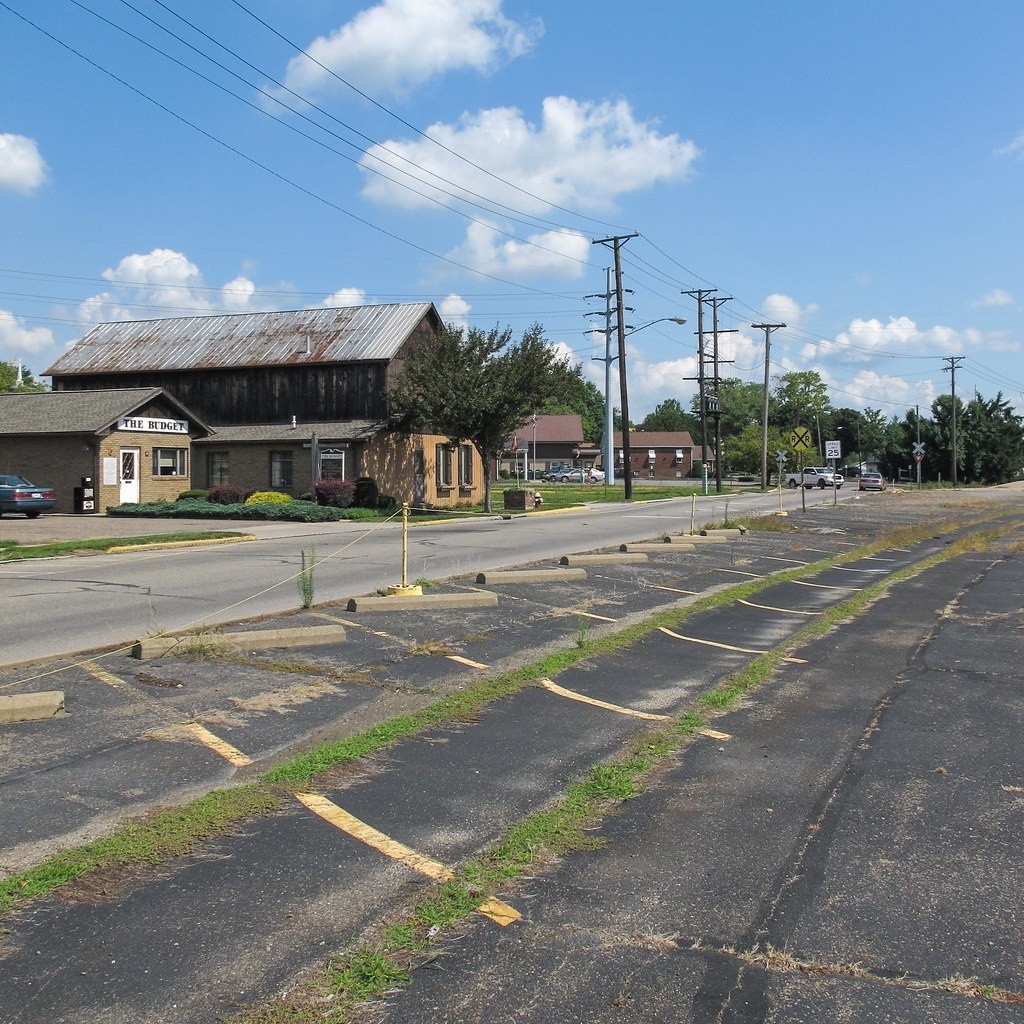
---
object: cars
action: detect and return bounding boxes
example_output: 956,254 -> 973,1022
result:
859,473 -> 887,491
0,475 -> 57,520
542,467 -> 606,484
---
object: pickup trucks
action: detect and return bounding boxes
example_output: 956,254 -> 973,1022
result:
784,467 -> 845,489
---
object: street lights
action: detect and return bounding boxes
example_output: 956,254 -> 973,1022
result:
617,316 -> 688,499
